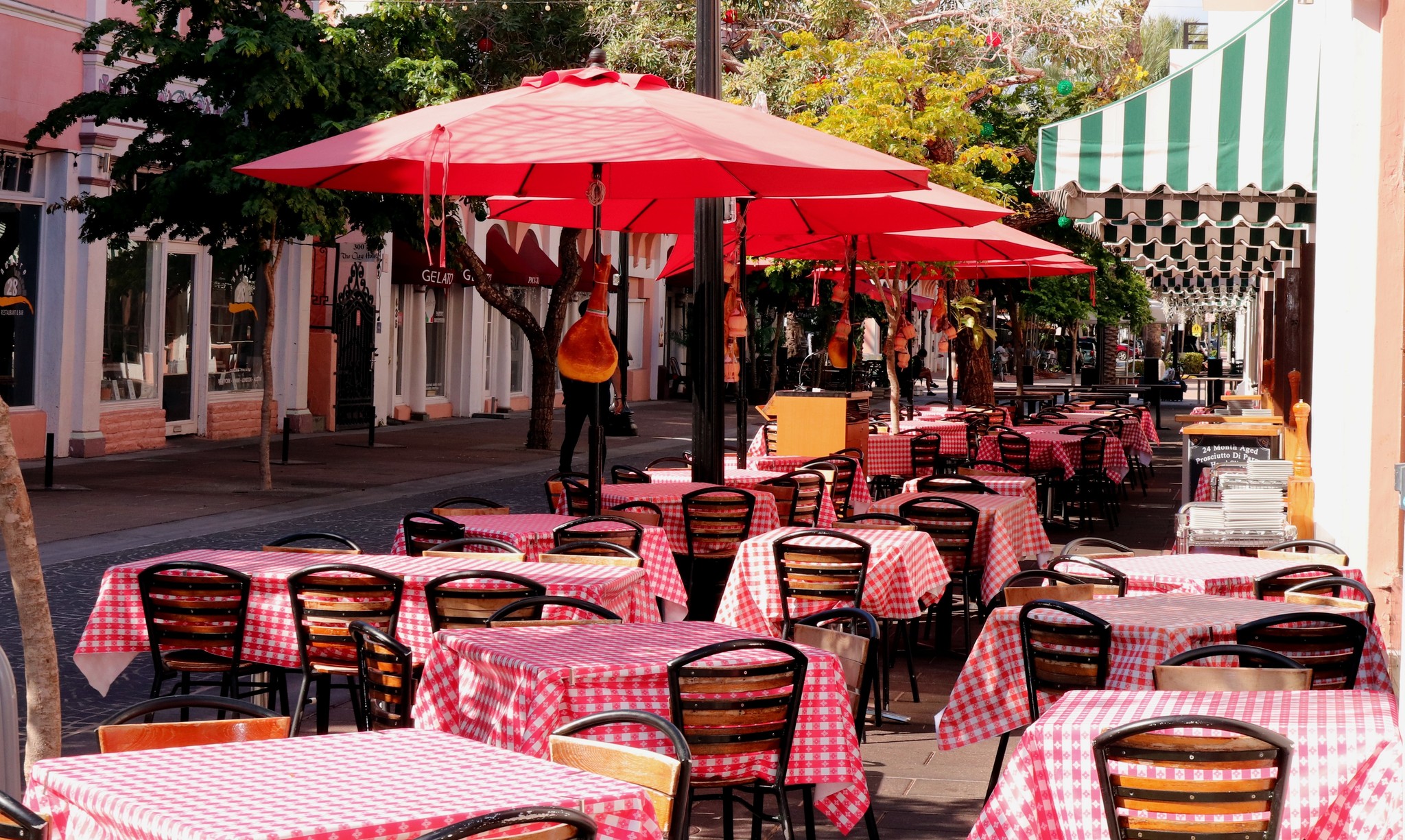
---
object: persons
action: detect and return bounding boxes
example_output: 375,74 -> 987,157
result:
1213,340 -> 1218,348
995,341 -> 1058,375
910,348 -> 938,396
558,298 -> 623,477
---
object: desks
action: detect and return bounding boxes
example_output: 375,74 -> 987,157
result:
763,384 -> 1181,534
554,483 -> 780,554
390,515 -> 688,622
73,549 -> 662,696
714,526 -> 952,710
1190,373 -> 1244,408
1042,553 -> 1389,669
862,492 -> 1053,606
627,469 -> 837,527
966,689 -> 1405,840
934,592 -> 1394,751
24,729 -> 665,840
411,621 -> 871,840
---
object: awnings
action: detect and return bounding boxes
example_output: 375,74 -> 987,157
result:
558,244 -> 595,291
1031,0 -> 1320,307
486,226 -> 540,287
442,227 -> 493,285
666,245 -> 694,288
518,233 -> 562,285
392,230 -> 454,286
585,245 -> 629,294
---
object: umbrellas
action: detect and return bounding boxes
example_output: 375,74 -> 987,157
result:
742,251 -> 1082,413
484,175 -> 1015,473
233,46 -> 934,513
685,222 -> 1071,391
806,263 -> 1098,411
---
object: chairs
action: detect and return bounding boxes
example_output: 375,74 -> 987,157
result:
670,357 -> 689,397
984,538 -> 1376,840
99,464 -> 986,840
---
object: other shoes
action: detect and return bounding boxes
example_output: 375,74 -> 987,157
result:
930,383 -> 939,388
927,391 -> 936,396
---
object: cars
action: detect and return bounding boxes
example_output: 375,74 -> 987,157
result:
1116,339 -> 1144,361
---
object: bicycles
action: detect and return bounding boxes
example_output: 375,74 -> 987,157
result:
990,351 -> 1005,382
1037,353 -> 1060,373
1164,349 -> 1191,379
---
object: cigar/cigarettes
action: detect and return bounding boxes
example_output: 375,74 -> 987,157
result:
617,413 -> 621,414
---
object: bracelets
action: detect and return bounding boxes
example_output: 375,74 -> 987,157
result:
615,398 -> 621,400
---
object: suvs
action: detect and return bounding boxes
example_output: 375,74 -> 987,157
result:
1055,336 -> 1097,374
1199,341 -> 1208,362
